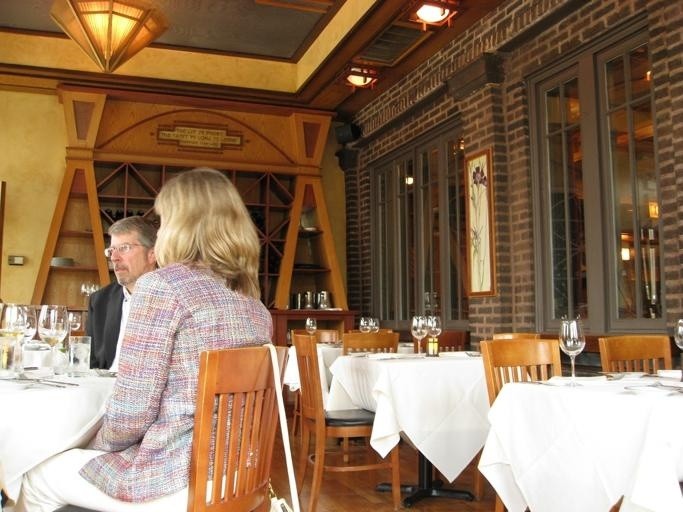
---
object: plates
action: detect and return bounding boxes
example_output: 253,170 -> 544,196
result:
465,351 -> 481,358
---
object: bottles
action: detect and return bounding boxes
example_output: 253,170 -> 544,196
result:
290,291 -> 331,309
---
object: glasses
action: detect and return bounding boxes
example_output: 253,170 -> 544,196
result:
104,243 -> 143,257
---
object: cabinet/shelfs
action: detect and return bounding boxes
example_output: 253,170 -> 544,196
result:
28,158 -> 349,311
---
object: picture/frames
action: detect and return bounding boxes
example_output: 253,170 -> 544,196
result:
461,148 -> 497,299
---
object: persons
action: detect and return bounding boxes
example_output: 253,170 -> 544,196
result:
2,168 -> 274,512
69,216 -> 160,370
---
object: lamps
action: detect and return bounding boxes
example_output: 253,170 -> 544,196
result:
342,65 -> 379,94
392,0 -> 467,33
48,1 -> 171,74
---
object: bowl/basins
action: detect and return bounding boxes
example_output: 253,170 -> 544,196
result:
49,256 -> 73,267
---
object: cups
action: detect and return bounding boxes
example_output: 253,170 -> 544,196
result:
359,316 -> 379,333
304,317 -> 317,335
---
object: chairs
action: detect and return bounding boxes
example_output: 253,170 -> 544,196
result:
0,315 -> 682,512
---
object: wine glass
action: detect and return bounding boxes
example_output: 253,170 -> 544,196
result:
672,318 -> 683,382
411,316 -> 428,360
559,320 -> 586,387
0,302 -> 91,387
427,315 -> 441,357
